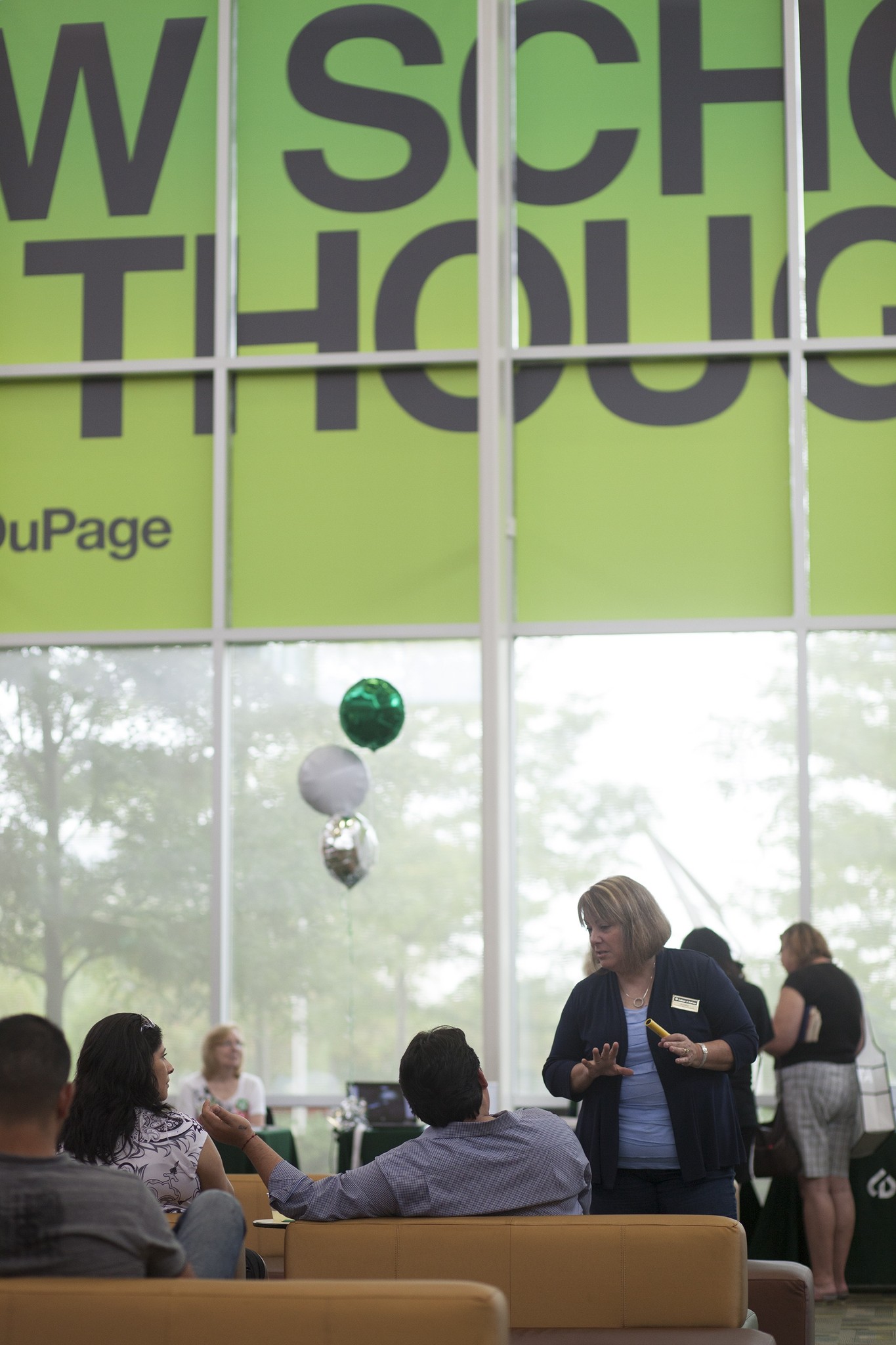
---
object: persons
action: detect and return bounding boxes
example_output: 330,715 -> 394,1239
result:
159,1023 -> 268,1133
540,875 -> 761,1220
761,921 -> 867,1302
680,927 -> 775,1258
200,1023 -> 594,1224
1,1012 -> 247,1280
57,1010 -> 269,1282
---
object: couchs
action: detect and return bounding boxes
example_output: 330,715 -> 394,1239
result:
0,1173 -> 815,1345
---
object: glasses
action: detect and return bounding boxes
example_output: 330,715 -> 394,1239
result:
140,1013 -> 155,1032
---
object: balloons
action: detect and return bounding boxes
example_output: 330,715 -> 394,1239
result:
321,809 -> 378,891
335,676 -> 403,751
295,742 -> 368,816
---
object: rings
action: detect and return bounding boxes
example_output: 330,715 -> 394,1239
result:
681,1048 -> 689,1056
594,1058 -> 602,1064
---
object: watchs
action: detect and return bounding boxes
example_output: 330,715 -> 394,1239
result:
694,1042 -> 708,1068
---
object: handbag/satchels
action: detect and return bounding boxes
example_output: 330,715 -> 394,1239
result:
744,1102 -> 802,1180
799,1004 -> 822,1044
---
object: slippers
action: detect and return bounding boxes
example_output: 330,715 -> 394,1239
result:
835,1289 -> 850,1301
814,1283 -> 838,1302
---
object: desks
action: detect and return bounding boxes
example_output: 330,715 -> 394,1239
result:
749,1129 -> 896,1295
210,1125 -> 301,1175
333,1125 -> 425,1175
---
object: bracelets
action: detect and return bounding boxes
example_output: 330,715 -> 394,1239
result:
241,1133 -> 259,1151
